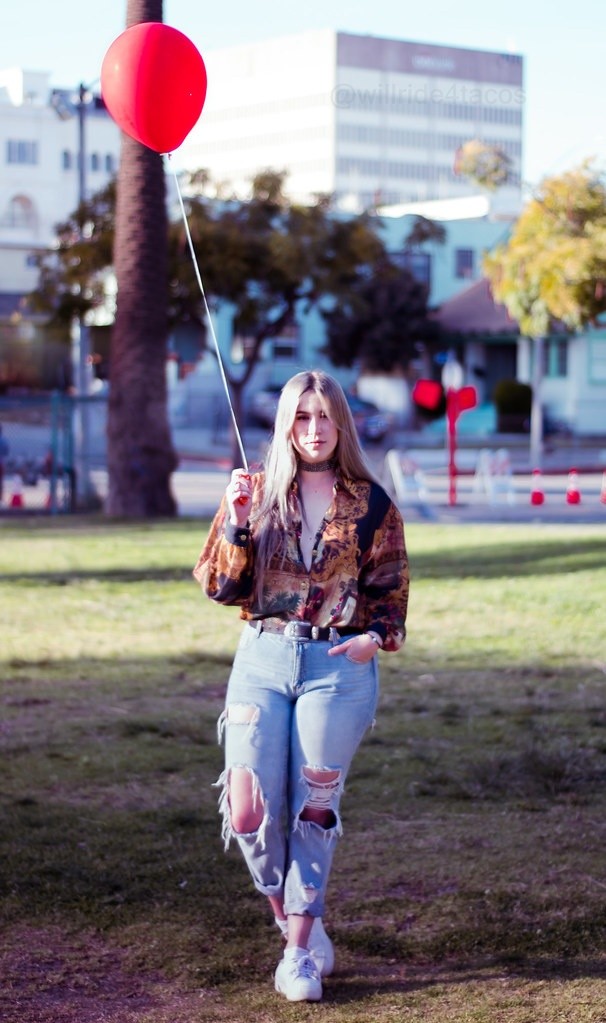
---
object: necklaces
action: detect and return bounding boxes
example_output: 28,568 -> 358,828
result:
296,456 -> 337,472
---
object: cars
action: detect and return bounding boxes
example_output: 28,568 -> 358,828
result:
254,380 -> 392,442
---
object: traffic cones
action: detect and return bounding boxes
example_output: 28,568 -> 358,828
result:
566,468 -> 579,505
530,468 -> 545,506
601,471 -> 606,504
12,473 -> 25,508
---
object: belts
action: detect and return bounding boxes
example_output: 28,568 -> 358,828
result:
248,620 -> 365,642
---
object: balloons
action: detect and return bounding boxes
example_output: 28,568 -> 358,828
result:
100,21 -> 208,152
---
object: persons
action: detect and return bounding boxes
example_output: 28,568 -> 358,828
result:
192,368 -> 410,1003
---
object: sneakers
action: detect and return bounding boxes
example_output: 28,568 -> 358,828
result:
274,911 -> 335,1001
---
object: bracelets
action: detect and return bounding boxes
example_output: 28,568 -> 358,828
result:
365,631 -> 381,647
